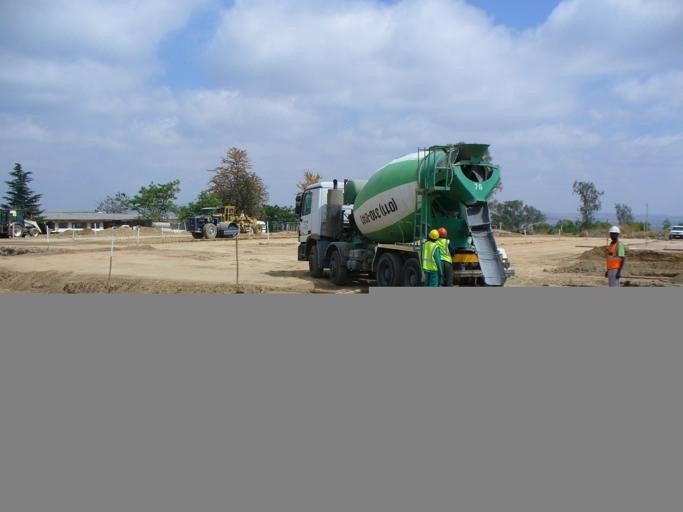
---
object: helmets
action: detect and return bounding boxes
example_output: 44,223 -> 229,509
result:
427,229 -> 439,241
607,225 -> 620,234
436,227 -> 448,238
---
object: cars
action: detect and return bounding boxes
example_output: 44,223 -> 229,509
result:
669,226 -> 683,240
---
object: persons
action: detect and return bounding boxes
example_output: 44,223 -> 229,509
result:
421,228 -> 444,287
603,225 -> 625,288
436,227 -> 455,286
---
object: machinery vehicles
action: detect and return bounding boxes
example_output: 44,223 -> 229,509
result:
296,143 -> 515,287
1,208 -> 42,237
187,206 -> 262,239
